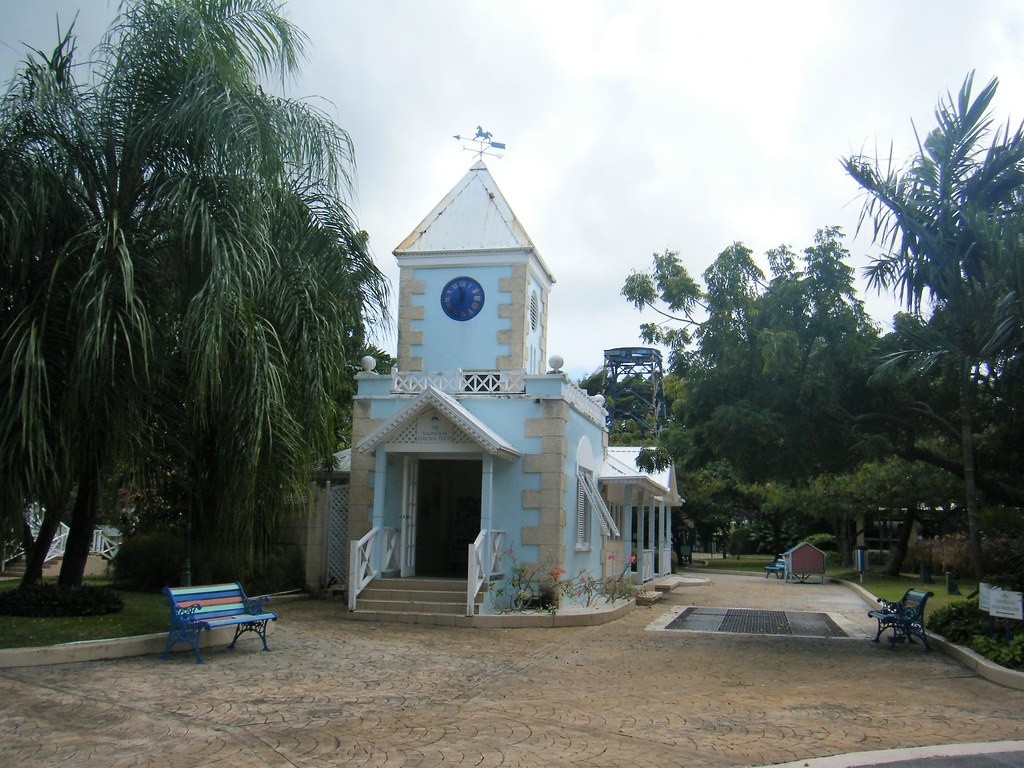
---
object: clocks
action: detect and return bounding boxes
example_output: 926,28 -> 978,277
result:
441,276 -> 485,321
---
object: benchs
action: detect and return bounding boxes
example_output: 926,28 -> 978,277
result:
867,588 -> 934,651
764,558 -> 785,579
160,582 -> 278,665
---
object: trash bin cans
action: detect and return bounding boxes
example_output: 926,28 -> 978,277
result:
853,546 -> 869,572
